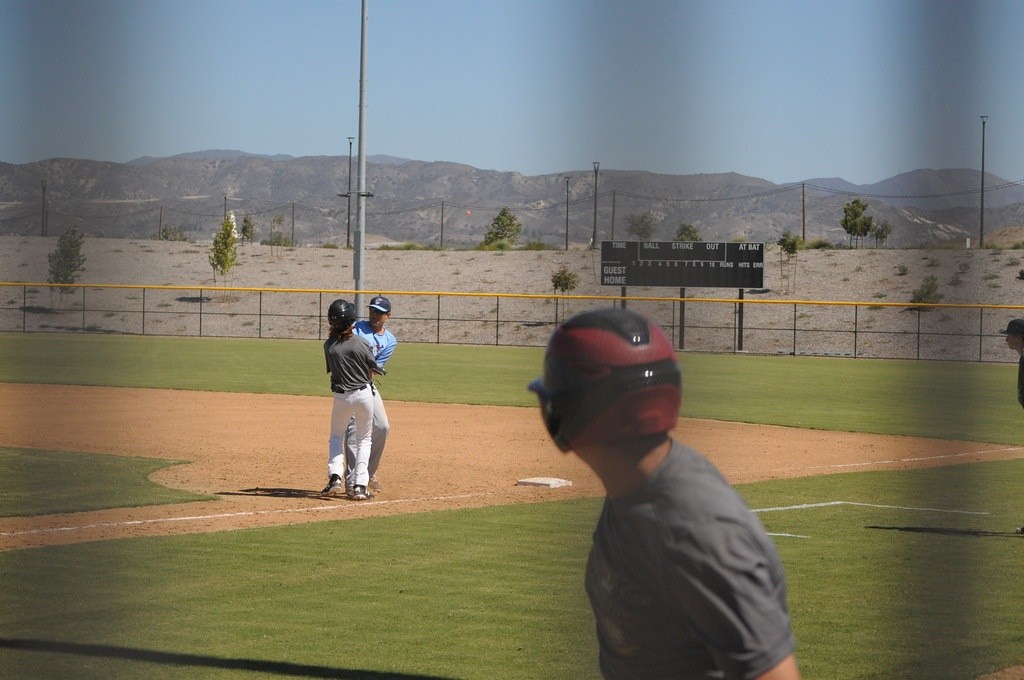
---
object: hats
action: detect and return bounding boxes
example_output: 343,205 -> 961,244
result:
366,297 -> 391,312
1000,319 -> 1024,335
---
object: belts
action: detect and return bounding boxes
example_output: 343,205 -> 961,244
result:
338,385 -> 367,393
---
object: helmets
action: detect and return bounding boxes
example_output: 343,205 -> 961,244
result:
528,307 -> 682,452
328,299 -> 356,329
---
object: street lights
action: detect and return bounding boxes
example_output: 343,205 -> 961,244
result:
593,160 -> 601,250
346,136 -> 356,249
564,176 -> 572,250
979,115 -> 989,248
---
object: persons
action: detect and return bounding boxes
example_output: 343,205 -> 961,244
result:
998,319 -> 1024,536
526,307 -> 803,680
322,296 -> 397,501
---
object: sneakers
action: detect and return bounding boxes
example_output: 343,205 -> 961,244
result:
369,475 -> 380,488
346,486 -> 355,497
320,474 -> 342,496
352,484 -> 373,500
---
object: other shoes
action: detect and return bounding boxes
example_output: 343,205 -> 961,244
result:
1016,528 -> 1024,534
